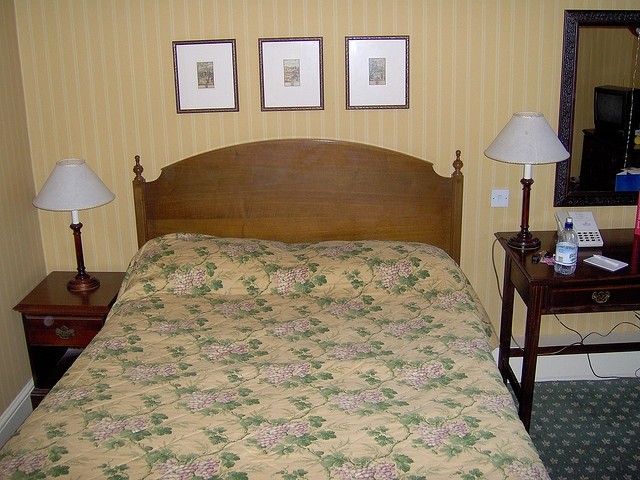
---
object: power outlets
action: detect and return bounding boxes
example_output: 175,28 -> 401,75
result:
490,189 -> 510,208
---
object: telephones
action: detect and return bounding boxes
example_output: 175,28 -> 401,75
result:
554,211 -> 604,248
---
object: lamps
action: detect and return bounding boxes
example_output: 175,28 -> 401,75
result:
483,110 -> 571,254
32,157 -> 116,295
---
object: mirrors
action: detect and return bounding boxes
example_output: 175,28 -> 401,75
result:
553,9 -> 640,206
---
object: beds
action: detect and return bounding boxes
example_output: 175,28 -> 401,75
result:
0,137 -> 551,479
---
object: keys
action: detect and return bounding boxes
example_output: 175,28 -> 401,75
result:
531,250 -> 545,264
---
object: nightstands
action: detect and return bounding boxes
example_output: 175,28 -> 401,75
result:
12,270 -> 126,411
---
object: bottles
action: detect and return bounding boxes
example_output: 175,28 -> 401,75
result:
554,217 -> 579,276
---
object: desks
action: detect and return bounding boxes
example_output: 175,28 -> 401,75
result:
494,228 -> 640,435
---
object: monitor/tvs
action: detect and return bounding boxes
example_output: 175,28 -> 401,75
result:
593,84 -> 640,131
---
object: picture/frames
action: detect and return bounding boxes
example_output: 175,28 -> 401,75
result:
344,34 -> 410,109
171,39 -> 240,114
258,36 -> 326,112
